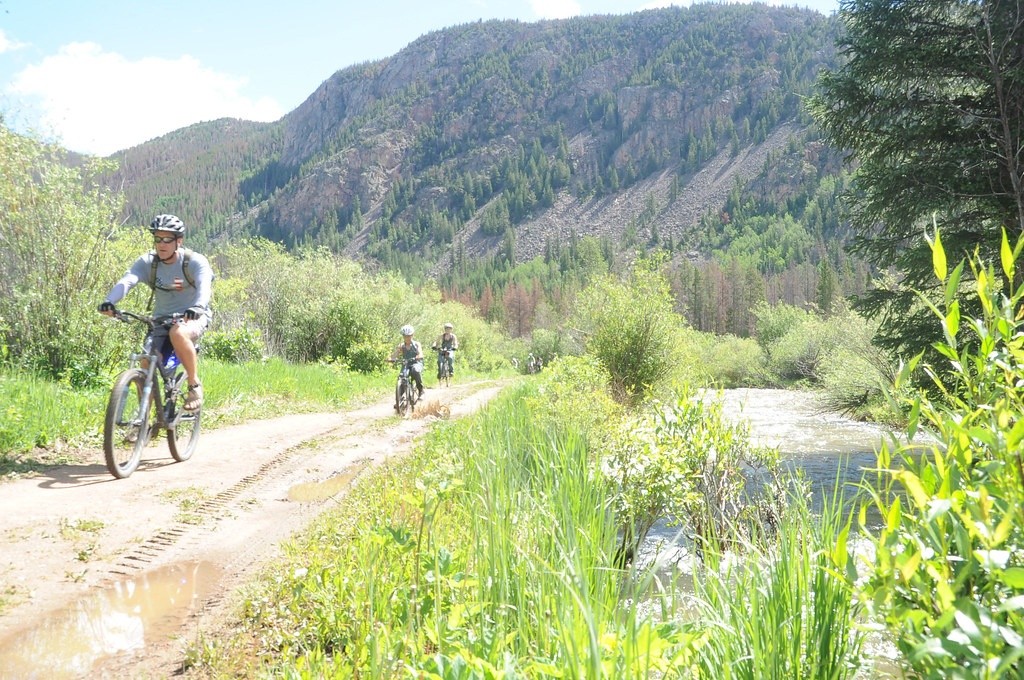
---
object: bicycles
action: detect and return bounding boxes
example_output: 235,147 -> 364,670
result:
434,346 -> 456,389
104,309 -> 202,480
388,357 -> 426,418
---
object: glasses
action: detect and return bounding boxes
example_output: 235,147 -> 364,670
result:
154,236 -> 178,244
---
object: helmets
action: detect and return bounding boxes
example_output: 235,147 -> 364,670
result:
444,323 -> 453,329
147,213 -> 186,238
399,325 -> 415,336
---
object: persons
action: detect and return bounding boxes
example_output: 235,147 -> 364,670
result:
386,325 -> 424,408
433,323 -> 457,379
511,353 -> 543,374
98,214 -> 213,443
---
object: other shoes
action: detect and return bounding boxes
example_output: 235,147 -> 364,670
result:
419,388 -> 424,396
393,404 -> 397,409
437,373 -> 442,379
449,371 -> 454,377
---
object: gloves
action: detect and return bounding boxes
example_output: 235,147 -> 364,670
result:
451,348 -> 456,351
431,347 -> 437,351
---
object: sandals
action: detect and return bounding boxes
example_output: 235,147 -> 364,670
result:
183,382 -> 203,412
128,427 -> 140,443
